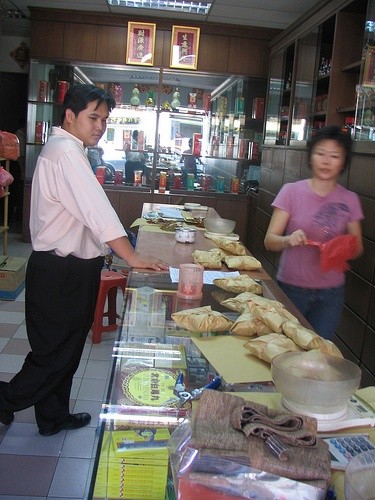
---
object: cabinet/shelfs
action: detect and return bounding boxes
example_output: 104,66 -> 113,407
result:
0,155 -> 10,256
247,0 -> 375,390
21,55 -> 267,245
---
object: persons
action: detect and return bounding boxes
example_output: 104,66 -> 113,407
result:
263,125 -> 364,340
0,83 -> 170,436
180,138 -> 203,183
125,130 -> 148,181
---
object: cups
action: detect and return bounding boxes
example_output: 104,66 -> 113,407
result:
177,264 -> 205,300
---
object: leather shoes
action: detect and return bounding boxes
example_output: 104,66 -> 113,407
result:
39,412 -> 91,436
0,380 -> 15,426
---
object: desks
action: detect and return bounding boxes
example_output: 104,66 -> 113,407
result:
145,162 -> 202,182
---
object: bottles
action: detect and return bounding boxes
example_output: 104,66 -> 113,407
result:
170,88 -> 180,109
130,84 -> 140,106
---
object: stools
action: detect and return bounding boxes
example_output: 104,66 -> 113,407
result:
90,270 -> 127,344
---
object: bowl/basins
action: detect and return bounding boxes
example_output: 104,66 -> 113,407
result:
344,449 -> 375,500
175,226 -> 197,244
269,350 -> 361,416
203,217 -> 236,234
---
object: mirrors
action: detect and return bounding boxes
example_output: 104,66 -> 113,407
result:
25,59 -> 245,191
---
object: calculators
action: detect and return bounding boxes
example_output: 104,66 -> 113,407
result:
324,435 -> 375,470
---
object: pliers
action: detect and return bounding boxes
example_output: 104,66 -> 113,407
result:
164,373 -> 220,409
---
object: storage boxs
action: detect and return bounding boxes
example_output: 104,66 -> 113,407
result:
37,79 -> 123,105
35,121 -> 46,143
107,128 -> 244,160
0,254 -> 29,300
186,91 -> 245,120
251,98 -> 265,120
97,165 -> 240,194
248,142 -> 259,160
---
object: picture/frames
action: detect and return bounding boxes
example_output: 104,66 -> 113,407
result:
125,22 -> 156,66
169,25 -> 201,71
